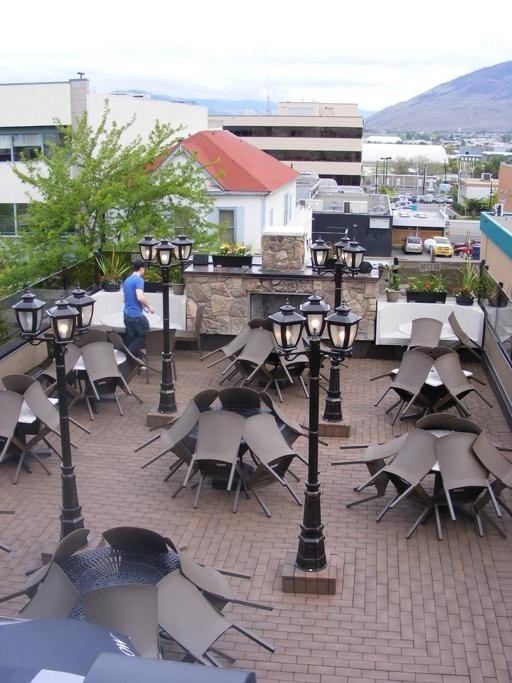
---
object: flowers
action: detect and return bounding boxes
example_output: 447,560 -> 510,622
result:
406,274 -> 446,293
212,243 -> 250,257
143,272 -> 162,283
453,287 -> 476,299
93,249 -> 131,281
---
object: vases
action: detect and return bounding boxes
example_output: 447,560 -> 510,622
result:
144,282 -> 160,293
172,268 -> 185,295
456,296 -> 474,306
102,279 -> 121,292
406,289 -> 447,304
211,255 -> 253,269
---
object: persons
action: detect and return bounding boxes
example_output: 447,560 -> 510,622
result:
124,259 -> 155,356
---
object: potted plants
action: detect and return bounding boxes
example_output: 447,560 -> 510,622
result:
381,264 -> 401,302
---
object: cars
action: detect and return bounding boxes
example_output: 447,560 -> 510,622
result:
390,190 -> 455,209
431,235 -> 452,257
454,241 -> 482,258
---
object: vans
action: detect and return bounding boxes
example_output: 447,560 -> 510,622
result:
401,235 -> 424,253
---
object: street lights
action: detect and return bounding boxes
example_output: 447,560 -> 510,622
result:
137,232 -> 193,412
271,291 -> 363,573
380,155 -> 393,187
311,231 -> 366,422
12,286 -> 97,550
